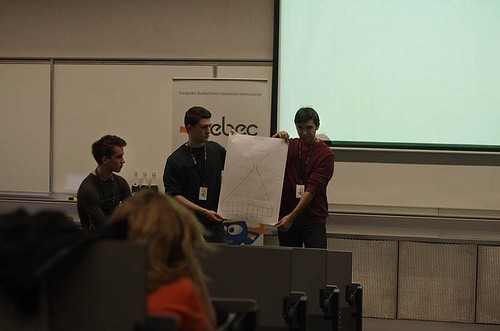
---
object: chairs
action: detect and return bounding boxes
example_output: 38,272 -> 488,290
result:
0,242 -> 363,331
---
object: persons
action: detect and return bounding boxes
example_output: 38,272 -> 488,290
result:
162,106 -> 239,244
0,188 -> 218,331
77,135 -> 133,240
271,107 -> 334,249
